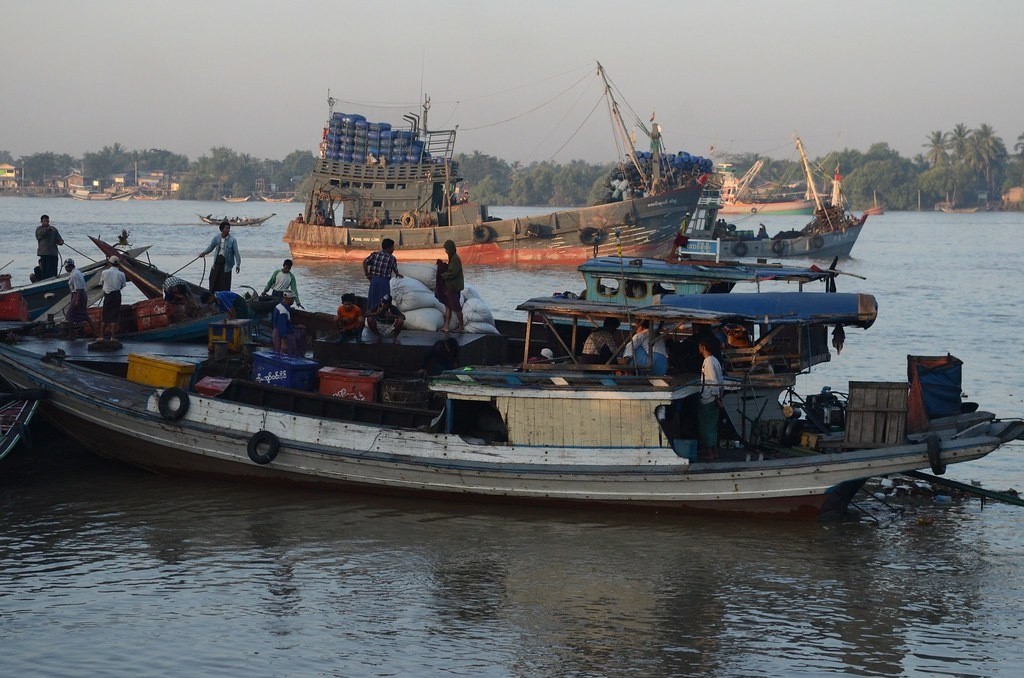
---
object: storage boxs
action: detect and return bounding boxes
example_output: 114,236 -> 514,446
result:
208,319 -> 252,354
0,292 -> 28,321
251,349 -> 320,393
840,381 -> 910,448
126,352 -> 196,391
316,366 -> 384,403
132,297 -> 169,332
84,304 -> 134,337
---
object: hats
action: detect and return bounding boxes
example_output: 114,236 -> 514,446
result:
381,294 -> 392,304
109,256 -> 119,264
61,258 -> 74,266
541,348 -> 555,364
283,290 -> 296,299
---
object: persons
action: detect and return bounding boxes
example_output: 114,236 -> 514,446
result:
199,222 -> 241,291
758,223 -> 765,237
435,240 -> 464,332
207,214 -> 212,218
273,291 -> 295,352
363,238 -> 404,309
61,258 -> 99,340
295,213 -> 303,223
200,291 -> 249,319
581,318 -> 624,375
313,206 -> 325,225
224,216 -> 227,221
682,323 -> 722,373
96,255 -> 126,341
696,337 -> 724,461
336,294 -> 365,344
438,185 -> 444,210
364,294 -> 405,344
30,214 -> 64,283
460,192 -> 468,202
717,219 -> 727,230
623,319 -> 668,376
262,259 -> 301,307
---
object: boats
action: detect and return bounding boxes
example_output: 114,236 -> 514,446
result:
222,194 -> 251,203
677,128 -> 870,262
1,242 -> 156,324
67,184 -> 135,201
133,192 -> 164,200
1,235 -> 1024,519
260,195 -> 296,203
942,186 -> 979,212
292,66 -> 713,267
194,211 -> 277,227
864,189 -> 885,215
719,160 -> 848,215
0,387 -> 52,464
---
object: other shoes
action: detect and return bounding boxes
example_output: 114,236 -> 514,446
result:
95,337 -> 104,341
392,338 -> 401,344
111,337 -> 119,341
356,338 -> 365,344
336,336 -> 344,344
372,336 -> 382,343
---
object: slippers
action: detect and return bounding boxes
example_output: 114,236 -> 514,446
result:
452,327 -> 464,333
437,328 -> 449,332
